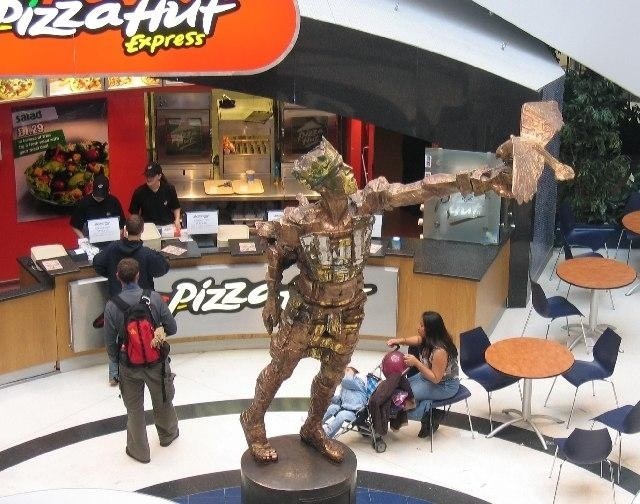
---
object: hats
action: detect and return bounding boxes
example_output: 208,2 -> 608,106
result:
142,161 -> 162,178
91,175 -> 109,198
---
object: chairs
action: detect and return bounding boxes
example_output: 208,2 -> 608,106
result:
549,428 -> 616,504
587,400 -> 640,484
459,326 -> 524,432
549,226 -> 615,281
406,344 -> 475,454
613,192 -> 640,264
544,326 -> 622,430
556,235 -> 616,310
521,268 -> 589,356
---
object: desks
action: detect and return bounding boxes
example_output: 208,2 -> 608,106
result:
555,257 -> 637,352
622,211 -> 640,295
484,336 -> 576,451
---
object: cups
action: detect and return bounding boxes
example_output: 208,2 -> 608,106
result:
240,170 -> 254,186
390,237 -> 402,252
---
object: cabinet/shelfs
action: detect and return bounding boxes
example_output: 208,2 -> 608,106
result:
210,88 -> 278,185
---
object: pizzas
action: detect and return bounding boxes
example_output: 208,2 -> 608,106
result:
0,79 -> 36,99
70,77 -> 102,92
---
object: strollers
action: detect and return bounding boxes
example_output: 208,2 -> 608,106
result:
333,339 -> 414,455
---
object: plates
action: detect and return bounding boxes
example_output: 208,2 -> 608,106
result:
25,141 -> 109,206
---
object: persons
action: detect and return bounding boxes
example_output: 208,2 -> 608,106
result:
129,162 -> 181,230
239,135 -> 515,463
387,312 -> 460,437
94,214 -> 170,385
321,366 -> 368,437
104,257 -> 179,462
69,174 -> 127,247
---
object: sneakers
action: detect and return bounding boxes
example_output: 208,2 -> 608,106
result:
416,409 -> 444,439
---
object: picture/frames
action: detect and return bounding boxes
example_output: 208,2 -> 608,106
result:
156,108 -> 211,166
0,78 -> 196,104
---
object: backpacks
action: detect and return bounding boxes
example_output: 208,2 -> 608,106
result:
108,287 -> 172,368
